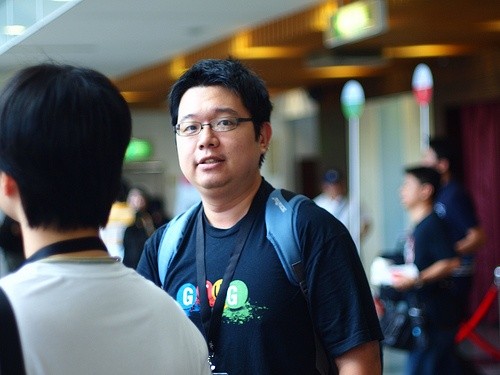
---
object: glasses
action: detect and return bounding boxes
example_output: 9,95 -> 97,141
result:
173,118 -> 253,136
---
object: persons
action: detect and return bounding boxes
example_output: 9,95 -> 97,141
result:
0,63 -> 213,375
310,168 -> 371,243
136,59 -> 385,375
392,165 -> 464,375
421,135 -> 486,375
99,179 -> 172,269
